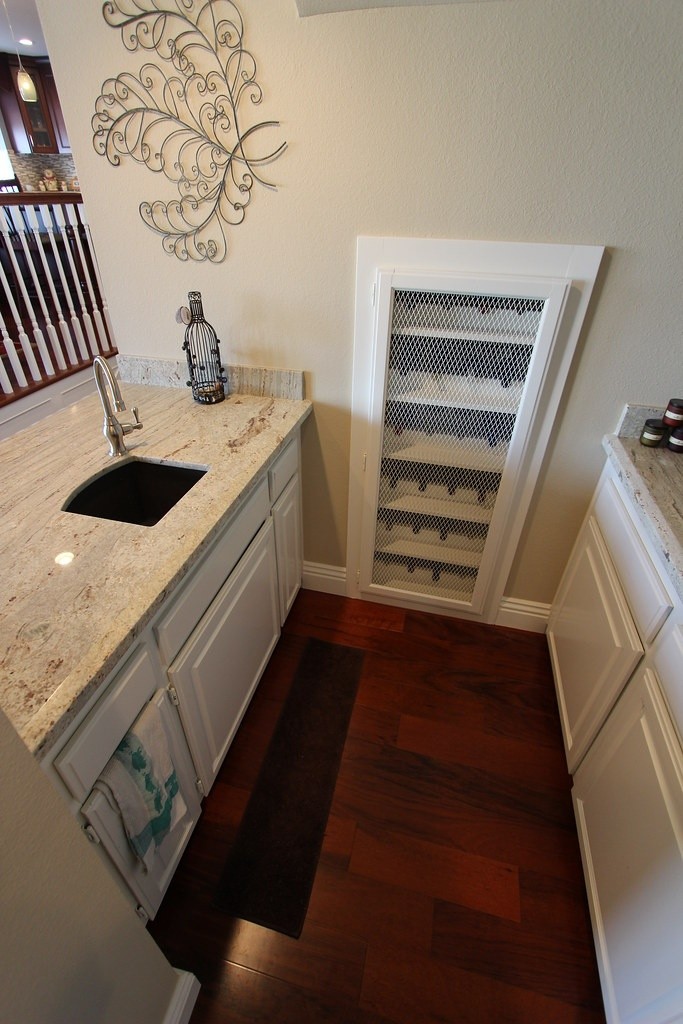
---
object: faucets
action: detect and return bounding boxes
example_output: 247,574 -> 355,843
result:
93,355 -> 144,458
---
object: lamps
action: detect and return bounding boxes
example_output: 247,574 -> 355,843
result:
2,0 -> 38,101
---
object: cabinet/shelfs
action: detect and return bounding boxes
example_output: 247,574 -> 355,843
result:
545,458 -> 683,1024
40,435 -> 307,925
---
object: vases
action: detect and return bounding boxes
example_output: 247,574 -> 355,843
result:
182,290 -> 225,405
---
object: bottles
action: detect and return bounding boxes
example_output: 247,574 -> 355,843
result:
640,419 -> 665,447
661,399 -> 683,427
666,427 -> 683,454
